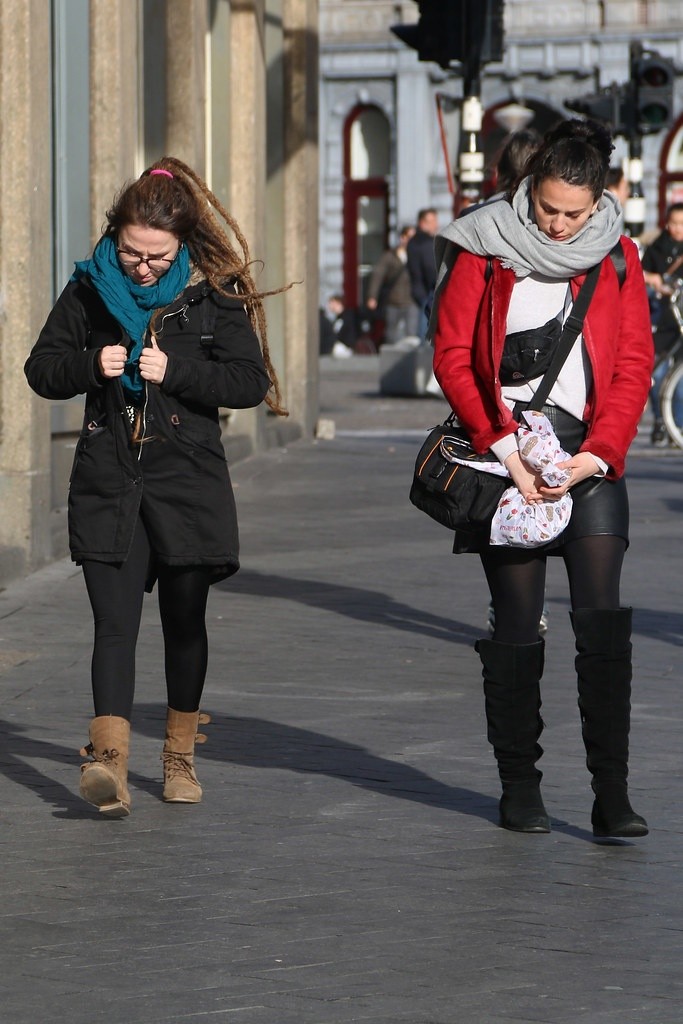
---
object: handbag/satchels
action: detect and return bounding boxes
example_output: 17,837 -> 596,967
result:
408,425 -> 515,533
499,282 -> 572,385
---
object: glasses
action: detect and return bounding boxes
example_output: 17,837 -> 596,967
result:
115,234 -> 182,273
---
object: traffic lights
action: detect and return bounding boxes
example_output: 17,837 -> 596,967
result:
634,59 -> 674,136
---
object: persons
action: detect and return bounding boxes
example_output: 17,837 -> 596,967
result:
604,168 -> 630,206
640,202 -> 683,447
23,157 -> 290,818
327,296 -> 357,358
457,130 -> 549,634
366,225 -> 418,342
407,208 -> 439,339
423,119 -> 655,838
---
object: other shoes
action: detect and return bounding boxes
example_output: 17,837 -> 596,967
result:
650,421 -> 669,447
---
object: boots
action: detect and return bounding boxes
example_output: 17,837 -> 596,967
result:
160,705 -> 210,803
569,605 -> 649,836
80,715 -> 132,819
475,633 -> 550,833
